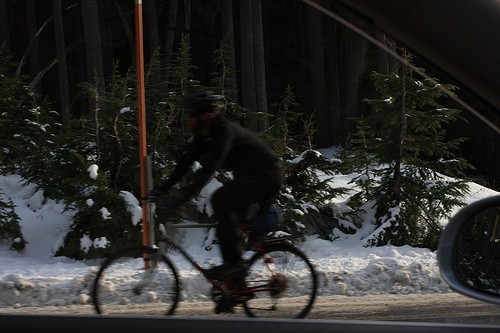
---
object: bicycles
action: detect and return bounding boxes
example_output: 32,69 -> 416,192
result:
89,195 -> 320,320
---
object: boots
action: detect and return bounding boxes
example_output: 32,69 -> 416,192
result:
214,269 -> 255,315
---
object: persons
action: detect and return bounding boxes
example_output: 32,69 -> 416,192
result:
148,86 -> 284,309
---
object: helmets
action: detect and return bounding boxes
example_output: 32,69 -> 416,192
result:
183,88 -> 227,120
